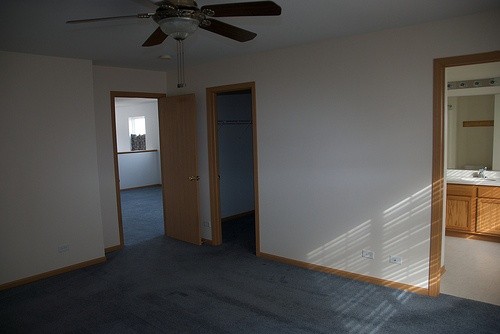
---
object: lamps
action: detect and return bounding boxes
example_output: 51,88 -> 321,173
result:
157,0 -> 202,89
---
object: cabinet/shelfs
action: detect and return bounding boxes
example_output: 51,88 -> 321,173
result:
445,183 -> 500,243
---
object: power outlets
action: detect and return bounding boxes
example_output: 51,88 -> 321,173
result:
362,249 -> 374,258
389,256 -> 402,264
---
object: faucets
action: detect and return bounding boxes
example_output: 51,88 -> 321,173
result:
478,167 -> 487,178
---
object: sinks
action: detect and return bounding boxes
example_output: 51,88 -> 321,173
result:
461,178 -> 497,182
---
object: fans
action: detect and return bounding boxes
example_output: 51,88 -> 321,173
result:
65,0 -> 282,47
